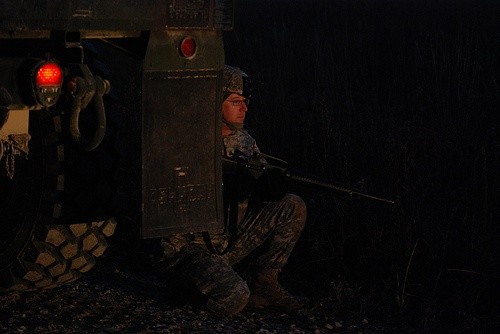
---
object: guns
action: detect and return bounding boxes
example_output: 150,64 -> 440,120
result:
220,146 -> 394,204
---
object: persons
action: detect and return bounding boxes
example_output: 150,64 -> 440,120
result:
159,65 -> 307,317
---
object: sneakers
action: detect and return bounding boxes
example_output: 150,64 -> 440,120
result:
249,278 -> 308,310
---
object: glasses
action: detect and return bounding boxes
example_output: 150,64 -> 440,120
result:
223,98 -> 250,106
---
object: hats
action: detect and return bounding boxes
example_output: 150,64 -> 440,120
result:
221,65 -> 251,95
